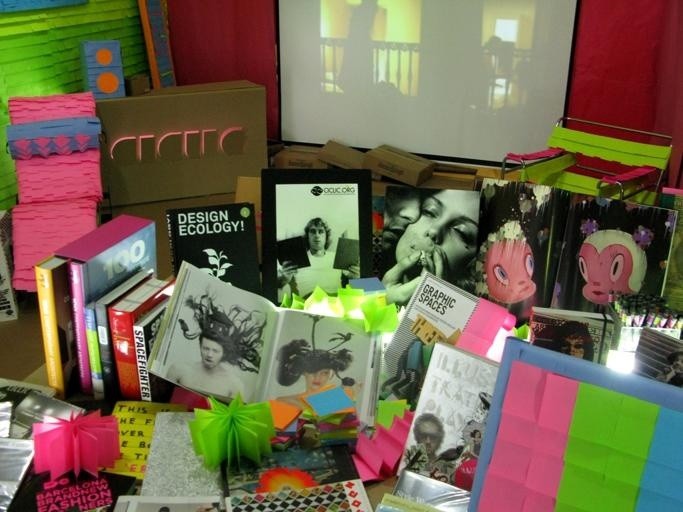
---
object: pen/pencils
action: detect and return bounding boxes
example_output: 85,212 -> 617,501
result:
608,289 -> 683,329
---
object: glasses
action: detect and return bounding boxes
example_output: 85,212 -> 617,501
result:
416,431 -> 439,443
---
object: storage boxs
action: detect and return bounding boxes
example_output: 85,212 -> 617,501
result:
96,80 -> 268,207
111,194 -> 261,278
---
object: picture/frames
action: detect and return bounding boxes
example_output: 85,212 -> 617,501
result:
261,168 -> 373,302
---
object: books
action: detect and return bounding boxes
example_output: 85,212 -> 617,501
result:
0,170 -> 682,512
